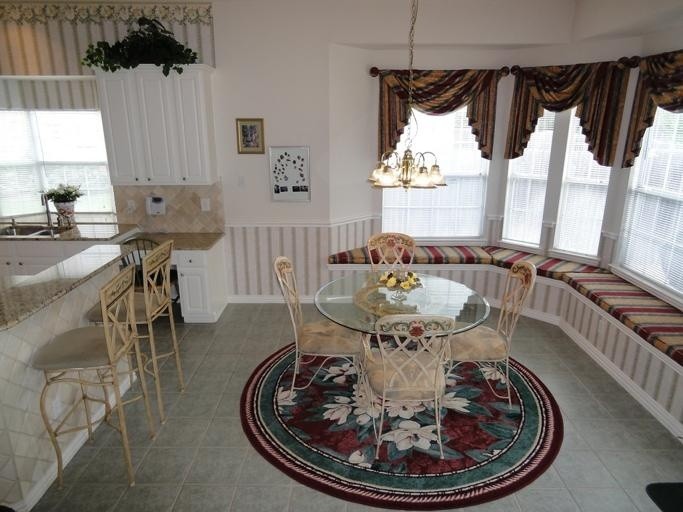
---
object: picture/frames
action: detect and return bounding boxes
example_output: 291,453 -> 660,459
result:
236,119 -> 265,154
269,145 -> 312,203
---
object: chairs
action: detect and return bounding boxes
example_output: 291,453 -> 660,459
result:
31,265 -> 154,489
273,256 -> 363,403
362,314 -> 454,461
86,239 -> 185,424
445,261 -> 536,410
367,232 -> 414,271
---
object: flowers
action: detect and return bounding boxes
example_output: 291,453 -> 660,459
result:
44,184 -> 83,203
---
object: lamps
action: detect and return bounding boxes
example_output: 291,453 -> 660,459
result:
368,0 -> 446,188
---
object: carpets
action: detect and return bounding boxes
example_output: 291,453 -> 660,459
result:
647,483 -> 683,512
240,341 -> 564,510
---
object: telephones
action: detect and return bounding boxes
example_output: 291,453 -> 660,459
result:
145,196 -> 165,215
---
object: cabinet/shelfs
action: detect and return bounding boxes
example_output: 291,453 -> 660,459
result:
95,64 -> 219,186
176,239 -> 227,324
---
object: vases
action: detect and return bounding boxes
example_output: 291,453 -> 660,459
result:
54,201 -> 74,218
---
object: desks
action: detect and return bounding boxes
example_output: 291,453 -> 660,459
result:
314,270 -> 490,350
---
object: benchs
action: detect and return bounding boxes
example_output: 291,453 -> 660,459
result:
328,246 -> 683,443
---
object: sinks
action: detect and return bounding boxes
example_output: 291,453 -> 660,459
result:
0,225 -> 44,241
31,226 -> 73,241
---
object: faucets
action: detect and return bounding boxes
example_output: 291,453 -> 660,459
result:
40,193 -> 53,226
